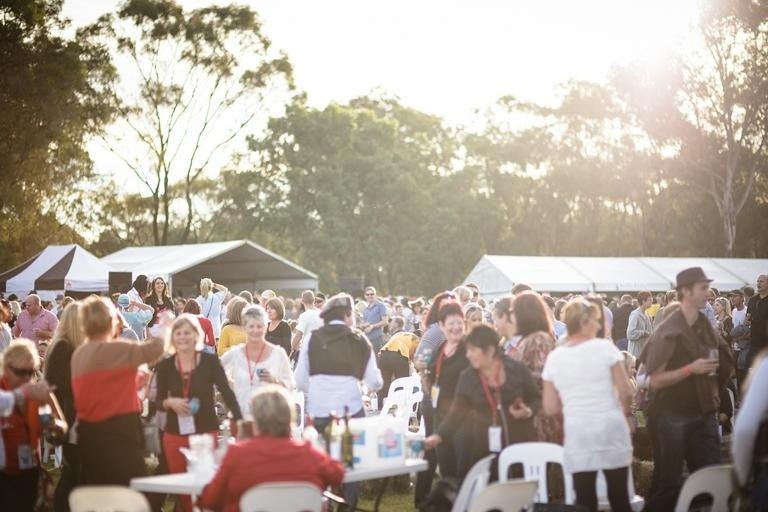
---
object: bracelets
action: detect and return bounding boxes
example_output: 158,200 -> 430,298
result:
625,411 -> 632,417
681,365 -> 691,377
14,389 -> 25,405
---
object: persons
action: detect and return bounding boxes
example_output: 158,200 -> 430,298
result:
1,267 -> 768,512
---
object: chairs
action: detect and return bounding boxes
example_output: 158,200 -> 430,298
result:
42,372 -> 741,512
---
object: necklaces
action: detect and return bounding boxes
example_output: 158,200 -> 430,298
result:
245,342 -> 267,386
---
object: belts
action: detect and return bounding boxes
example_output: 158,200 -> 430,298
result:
707,349 -> 720,377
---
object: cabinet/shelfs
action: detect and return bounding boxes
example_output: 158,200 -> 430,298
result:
370,389 -> 378,416
304,405 -> 354,465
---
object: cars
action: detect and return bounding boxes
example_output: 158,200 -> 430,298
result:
421,346 -> 432,367
256,368 -> 265,380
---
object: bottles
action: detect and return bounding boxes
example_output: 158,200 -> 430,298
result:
319,292 -> 354,317
728,289 -> 743,296
673,268 -> 713,288
55,295 -> 63,300
118,294 -> 130,308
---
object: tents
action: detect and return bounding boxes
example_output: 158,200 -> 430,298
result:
462,255 -> 768,307
99,239 -> 319,315
1,244 -> 133,316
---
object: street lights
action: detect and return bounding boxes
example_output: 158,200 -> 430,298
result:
135,414 -> 161,454
34,440 -> 65,512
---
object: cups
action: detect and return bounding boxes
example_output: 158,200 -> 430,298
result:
8,363 -> 36,377
